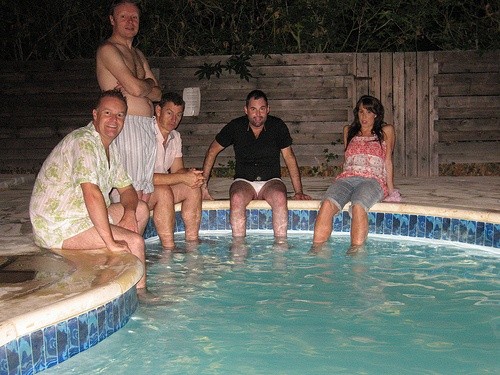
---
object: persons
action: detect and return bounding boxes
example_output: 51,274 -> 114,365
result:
304,97 -> 402,256
96,0 -> 163,199
200,90 -> 313,257
141,90 -> 207,253
29,90 -> 150,304
